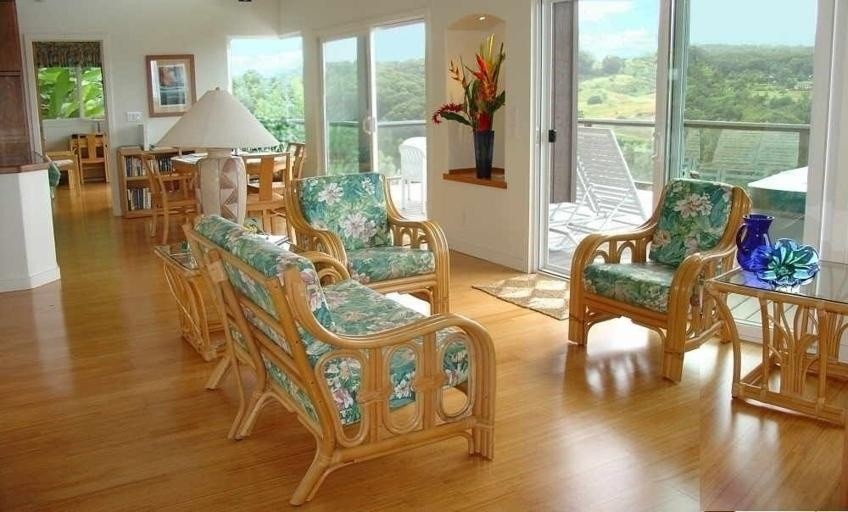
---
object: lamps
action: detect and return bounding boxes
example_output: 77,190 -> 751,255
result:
151,86 -> 279,228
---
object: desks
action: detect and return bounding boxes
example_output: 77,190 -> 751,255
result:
702,259 -> 848,425
68,134 -> 108,185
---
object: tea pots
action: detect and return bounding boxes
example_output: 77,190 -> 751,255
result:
735,213 -> 775,273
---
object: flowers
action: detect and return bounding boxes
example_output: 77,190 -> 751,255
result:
432,39 -> 507,131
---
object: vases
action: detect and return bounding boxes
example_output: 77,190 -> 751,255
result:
473,132 -> 495,181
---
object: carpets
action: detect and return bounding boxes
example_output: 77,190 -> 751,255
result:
470,270 -> 570,320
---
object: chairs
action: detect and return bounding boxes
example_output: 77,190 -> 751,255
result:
44,148 -> 84,200
182,171 -> 496,507
78,132 -> 110,184
136,141 -> 307,246
568,177 -> 752,382
547,127 -> 650,247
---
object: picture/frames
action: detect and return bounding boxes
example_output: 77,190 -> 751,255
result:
144,53 -> 197,119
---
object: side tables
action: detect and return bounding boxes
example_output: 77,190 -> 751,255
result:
152,240 -> 306,364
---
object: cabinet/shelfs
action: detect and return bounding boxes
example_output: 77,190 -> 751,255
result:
116,146 -> 199,220
